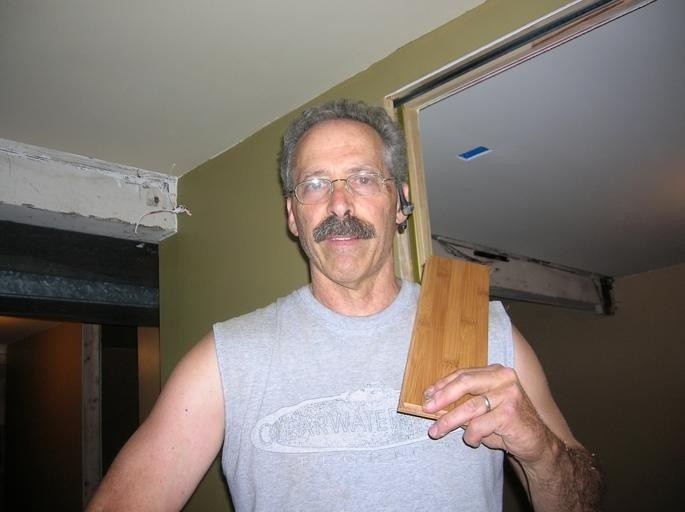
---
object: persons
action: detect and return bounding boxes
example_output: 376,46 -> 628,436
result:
88,101 -> 607,512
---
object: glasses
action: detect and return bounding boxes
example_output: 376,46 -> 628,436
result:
288,172 -> 397,205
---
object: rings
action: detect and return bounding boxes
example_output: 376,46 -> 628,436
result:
479,394 -> 490,411
